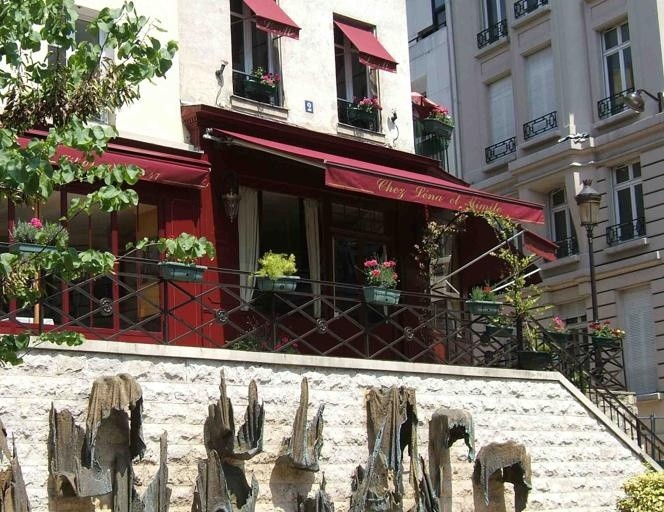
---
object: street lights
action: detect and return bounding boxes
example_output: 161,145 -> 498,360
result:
573,178 -> 605,387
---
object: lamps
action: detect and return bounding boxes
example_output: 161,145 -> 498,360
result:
622,89 -> 664,114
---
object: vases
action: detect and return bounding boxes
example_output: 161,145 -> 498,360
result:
363,286 -> 403,305
545,330 -> 571,344
465,300 -> 503,315
8,242 -> 61,260
592,337 -> 621,348
424,119 -> 455,139
348,109 -> 378,122
244,80 -> 276,98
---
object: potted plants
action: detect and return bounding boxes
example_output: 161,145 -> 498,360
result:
254,250 -> 301,293
518,322 -> 550,370
125,232 -> 216,283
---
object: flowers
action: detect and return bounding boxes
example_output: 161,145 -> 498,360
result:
362,253 -> 402,289
543,314 -> 571,332
252,66 -> 281,88
350,94 -> 382,114
589,319 -> 626,339
8,218 -> 70,246
427,104 -> 453,126
467,280 -> 499,301
